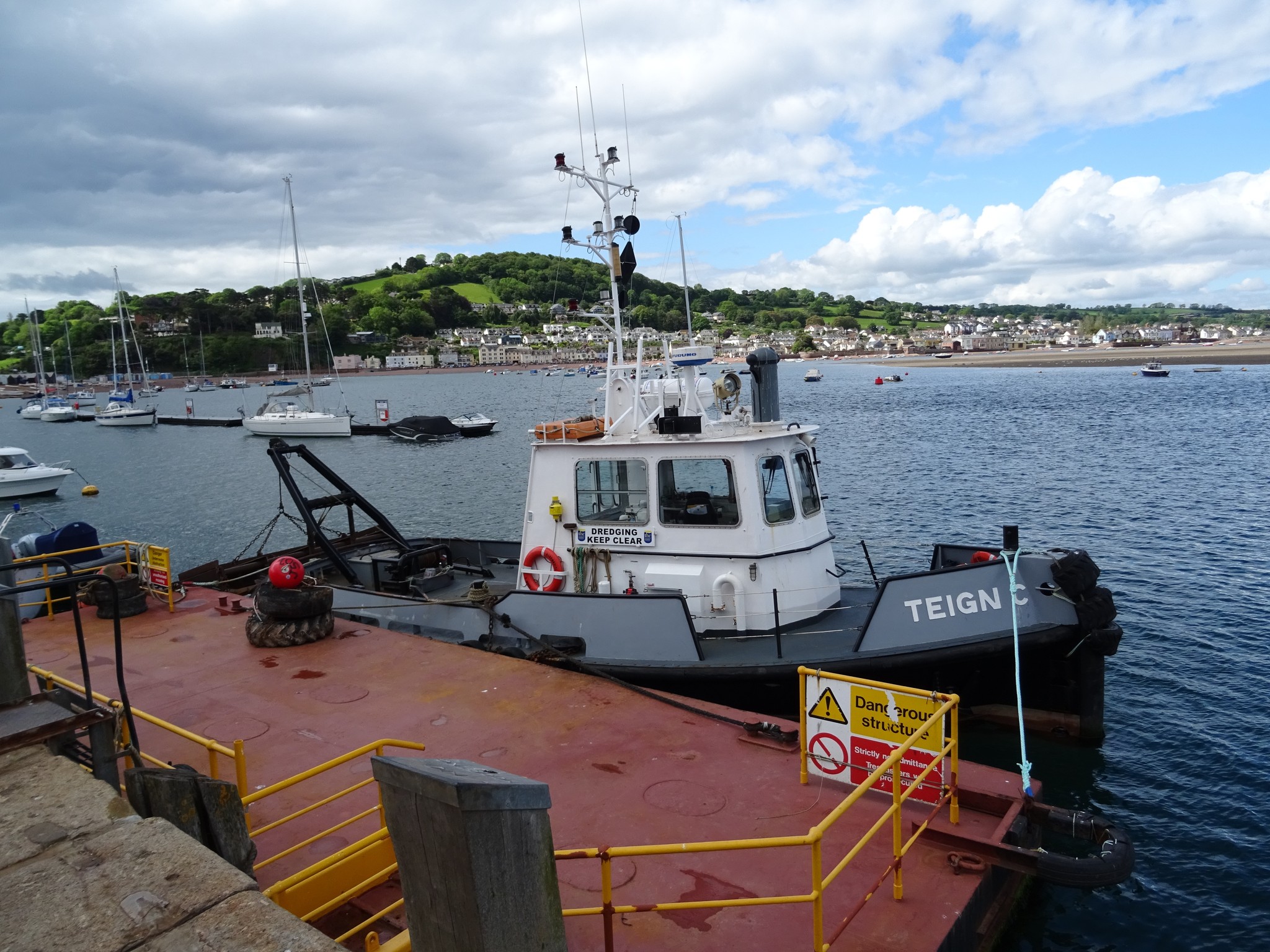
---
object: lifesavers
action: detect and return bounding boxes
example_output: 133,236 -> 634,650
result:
244,610 -> 337,649
522,544 -> 562,592
255,580 -> 333,625
970,551 -> 997,564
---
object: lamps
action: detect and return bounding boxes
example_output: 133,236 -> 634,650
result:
599,287 -> 609,301
614,215 -> 626,229
554,152 -> 568,168
561,225 -> 575,242
593,221 -> 603,234
712,373 -> 742,414
607,146 -> 620,161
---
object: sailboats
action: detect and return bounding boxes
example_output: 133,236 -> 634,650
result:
17,265 -> 164,428
199,331 -> 216,391
319,350 -> 337,382
240,174 -> 356,439
183,338 -> 198,392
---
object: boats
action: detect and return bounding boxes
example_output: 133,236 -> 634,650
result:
303,382 -> 331,387
803,368 -> 824,382
711,359 -> 729,365
720,366 -> 737,374
0,446 -> 74,501
734,359 -> 738,361
885,374 -> 903,382
386,413 -> 499,443
172,2 -> 1128,811
516,372 -> 523,375
623,358 -> 684,379
483,370 -> 494,373
779,340 -> 1244,377
219,373 -> 251,389
529,361 -> 607,378
0,502 -> 139,621
258,370 -> 298,386
698,366 -> 707,375
738,369 -> 751,375
500,369 -> 512,375
